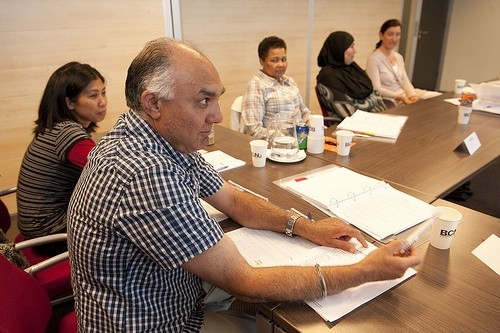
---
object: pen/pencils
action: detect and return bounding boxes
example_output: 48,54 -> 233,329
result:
215,165 -> 229,172
399,213 -> 439,254
350,130 -> 376,135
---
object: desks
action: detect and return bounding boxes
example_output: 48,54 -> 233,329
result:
91,92 -> 500,333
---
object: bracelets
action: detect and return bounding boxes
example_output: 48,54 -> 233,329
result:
315,264 -> 327,296
286,214 -> 302,237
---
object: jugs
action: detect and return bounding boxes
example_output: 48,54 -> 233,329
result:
266,111 -> 299,161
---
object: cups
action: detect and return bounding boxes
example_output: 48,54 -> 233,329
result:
249,140 -> 267,168
454,80 -> 466,93
335,130 -> 353,155
306,115 -> 325,154
457,105 -> 472,124
430,206 -> 461,250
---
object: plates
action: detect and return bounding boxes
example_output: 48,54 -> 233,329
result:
267,149 -> 307,163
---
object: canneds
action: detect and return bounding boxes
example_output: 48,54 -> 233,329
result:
296,123 -> 309,150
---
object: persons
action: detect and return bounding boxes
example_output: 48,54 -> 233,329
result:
67,37 -> 421,333
16,62 -> 107,257
317,31 -> 388,125
365,18 -> 442,108
241,36 -> 327,141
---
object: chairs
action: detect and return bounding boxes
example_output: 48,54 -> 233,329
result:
315,86 -> 396,127
0,188 -> 78,333
